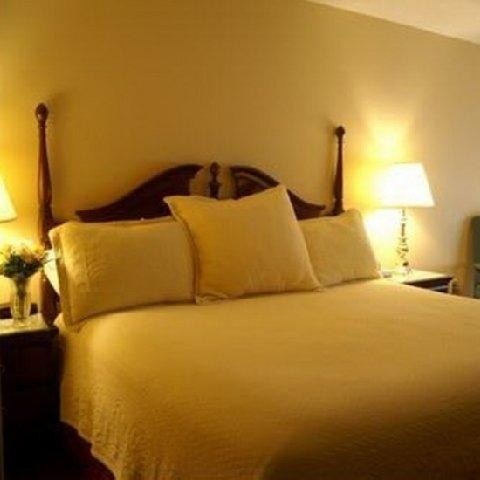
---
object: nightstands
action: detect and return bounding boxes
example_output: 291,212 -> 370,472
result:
382,269 -> 456,295
1,315 -> 61,469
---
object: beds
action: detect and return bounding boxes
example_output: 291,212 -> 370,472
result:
34,100 -> 479,480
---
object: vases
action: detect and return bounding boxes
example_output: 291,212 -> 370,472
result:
9,276 -> 33,327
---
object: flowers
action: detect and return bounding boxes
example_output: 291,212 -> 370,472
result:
1,241 -> 52,277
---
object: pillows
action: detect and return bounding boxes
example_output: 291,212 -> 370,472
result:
46,216 -> 197,334
161,180 -> 325,305
297,207 -> 382,288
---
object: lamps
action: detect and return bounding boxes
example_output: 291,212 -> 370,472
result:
0,173 -> 19,224
380,161 -> 438,267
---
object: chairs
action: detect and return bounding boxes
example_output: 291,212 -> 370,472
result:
465,215 -> 480,298
